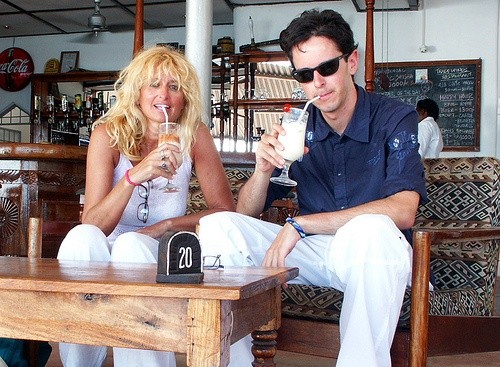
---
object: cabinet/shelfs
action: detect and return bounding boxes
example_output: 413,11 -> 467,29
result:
226,51 -> 309,141
28,71 -> 123,145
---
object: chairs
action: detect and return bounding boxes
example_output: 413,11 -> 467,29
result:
260,158 -> 500,367
27,168 -> 256,259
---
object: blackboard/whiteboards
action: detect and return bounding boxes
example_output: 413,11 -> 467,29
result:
373,58 -> 482,151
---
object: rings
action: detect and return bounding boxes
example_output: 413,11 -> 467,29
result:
159,146 -> 166,169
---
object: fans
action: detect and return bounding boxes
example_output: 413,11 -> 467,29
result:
42,0 -> 166,37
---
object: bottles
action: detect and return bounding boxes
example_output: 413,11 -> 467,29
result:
48,90 -> 105,144
4,49 -> 14,87
256,126 -> 266,137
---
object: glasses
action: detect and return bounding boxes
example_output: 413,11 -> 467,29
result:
203,255 -> 224,270
292,51 -> 350,82
136,180 -> 150,224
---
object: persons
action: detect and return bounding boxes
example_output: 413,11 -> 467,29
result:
196,7 -> 425,367
56,45 -> 232,367
413,99 -> 443,159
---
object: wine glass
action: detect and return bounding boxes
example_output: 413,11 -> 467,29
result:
240,86 -> 305,98
270,106 -> 310,187
159,122 -> 185,193
217,90 -> 230,104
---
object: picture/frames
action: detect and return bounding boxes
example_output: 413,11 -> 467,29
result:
59,50 -> 80,73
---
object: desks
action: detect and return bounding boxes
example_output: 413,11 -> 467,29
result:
0,256 -> 299,367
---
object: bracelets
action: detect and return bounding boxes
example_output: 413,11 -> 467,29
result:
285,217 -> 306,238
125,169 -> 140,186
